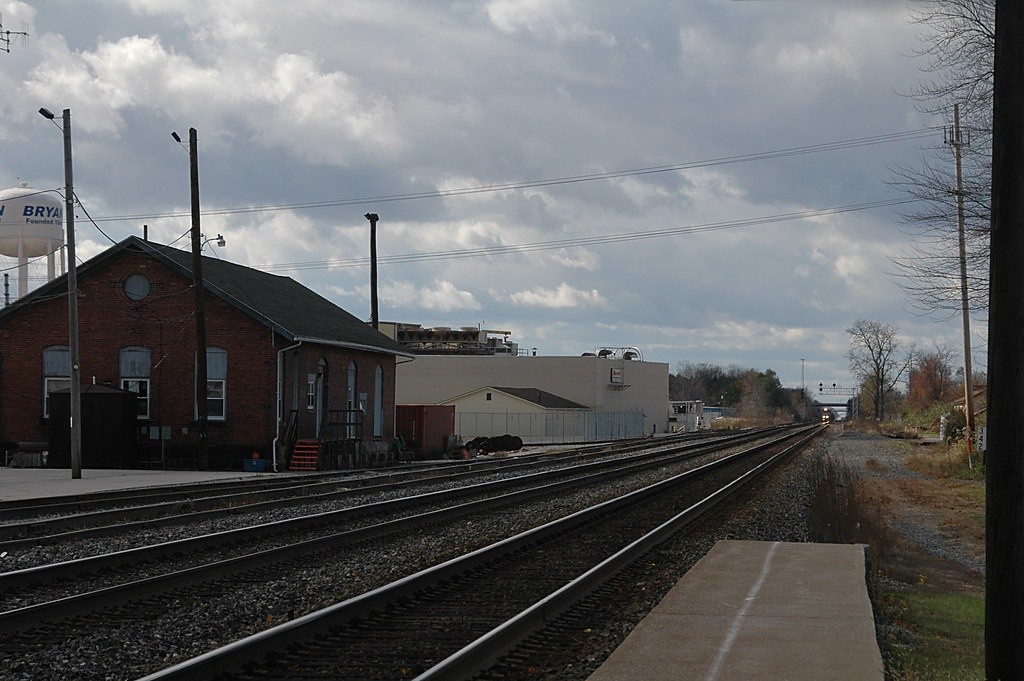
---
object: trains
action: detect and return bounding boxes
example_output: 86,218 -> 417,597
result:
822,407 -> 840,424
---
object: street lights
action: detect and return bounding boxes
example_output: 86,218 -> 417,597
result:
38,106 -> 83,479
191,234 -> 226,470
